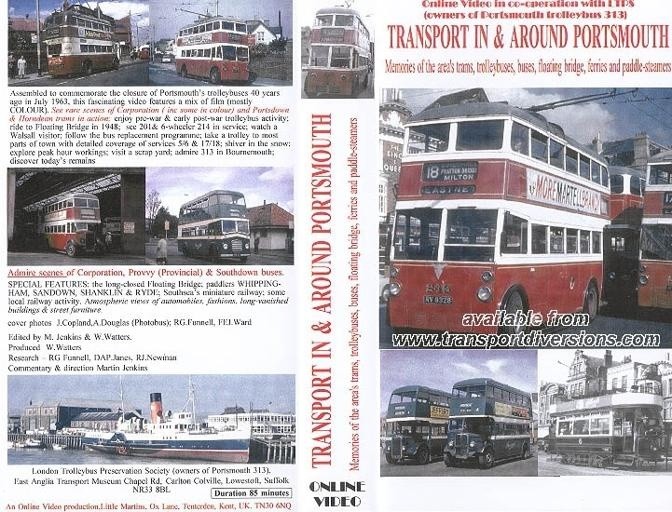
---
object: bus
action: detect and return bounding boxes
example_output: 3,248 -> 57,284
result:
175,21 -> 249,83
544,404 -> 667,467
176,190 -> 251,263
303,1 -> 374,97
445,376 -> 533,468
41,194 -> 102,259
40,4 -> 120,79
384,385 -> 454,464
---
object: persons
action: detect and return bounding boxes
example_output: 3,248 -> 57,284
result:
8,52 -> 17,80
154,234 -> 167,265
253,231 -> 260,254
104,232 -> 112,248
17,55 -> 28,80
129,49 -> 137,64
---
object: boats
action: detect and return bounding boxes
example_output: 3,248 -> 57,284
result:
15,438 -> 66,450
85,376 -> 271,464
